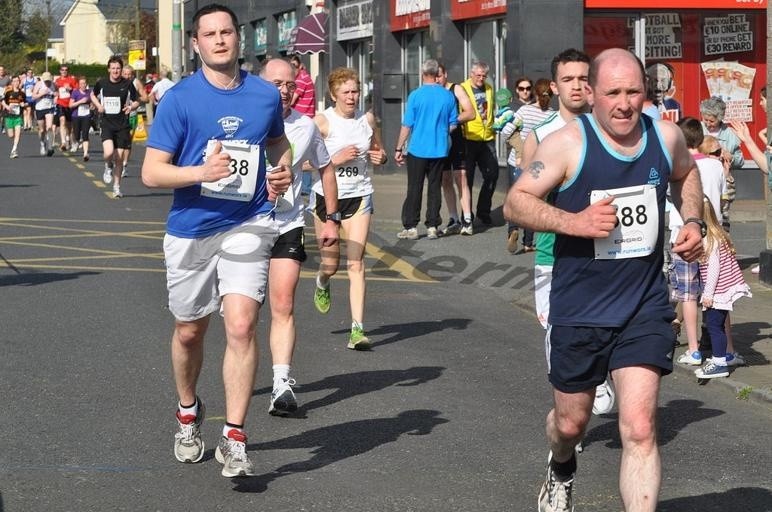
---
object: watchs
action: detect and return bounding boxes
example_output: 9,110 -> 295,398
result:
327,211 -> 341,223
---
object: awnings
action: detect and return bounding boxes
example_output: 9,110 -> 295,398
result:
293,12 -> 329,55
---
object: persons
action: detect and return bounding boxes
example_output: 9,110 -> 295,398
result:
141,5 -> 294,478
290,55 -> 316,117
521,50 -> 614,451
258,59 -> 341,416
395,59 -> 499,239
503,49 -> 708,512
730,86 -> 772,191
303,67 -> 389,349
493,77 -> 555,253
0,56 -> 175,197
648,63 -> 753,378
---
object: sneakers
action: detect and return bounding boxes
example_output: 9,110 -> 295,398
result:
677,349 -> 746,379
214,429 -> 256,478
103,161 -> 113,184
174,396 -> 205,463
347,329 -> 370,350
508,230 -> 518,252
314,284 -> 331,314
268,379 -> 299,418
113,189 -> 122,198
537,452 -> 574,512
10,152 -> 18,159
397,218 -> 473,240
121,166 -> 128,177
591,379 -> 614,417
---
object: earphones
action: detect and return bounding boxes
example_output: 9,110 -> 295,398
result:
238,40 -> 241,47
194,44 -> 199,50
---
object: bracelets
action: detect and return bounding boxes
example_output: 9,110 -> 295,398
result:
382,157 -> 388,164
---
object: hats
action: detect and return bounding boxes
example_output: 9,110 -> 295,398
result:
496,89 -> 512,106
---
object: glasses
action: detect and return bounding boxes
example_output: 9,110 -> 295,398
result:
517,86 -> 531,91
